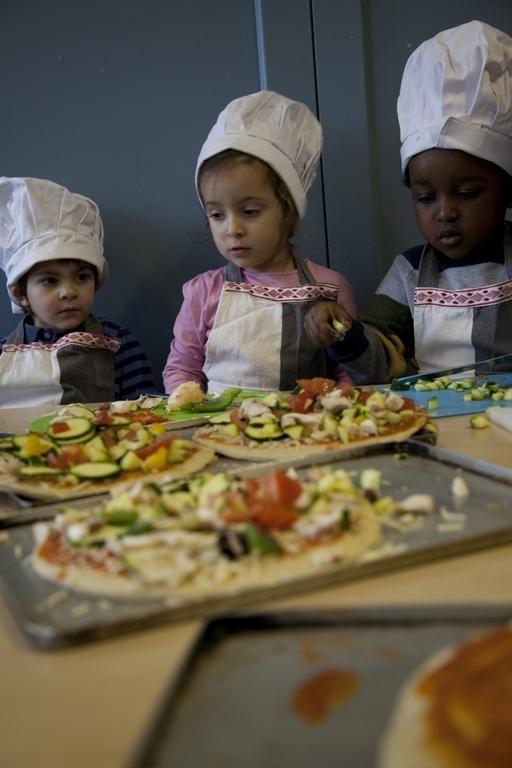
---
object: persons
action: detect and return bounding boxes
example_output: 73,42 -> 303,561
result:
302,147 -> 511,385
0,259 -> 163,404
160,149 -> 357,398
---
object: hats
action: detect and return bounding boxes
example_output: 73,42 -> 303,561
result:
397,20 -> 512,182
195,90 -> 323,220
0,175 -> 108,313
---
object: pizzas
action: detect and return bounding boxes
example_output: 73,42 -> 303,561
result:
0,405 -> 215,499
378,624 -> 511,768
191,379 -> 427,461
29,471 -> 383,596
